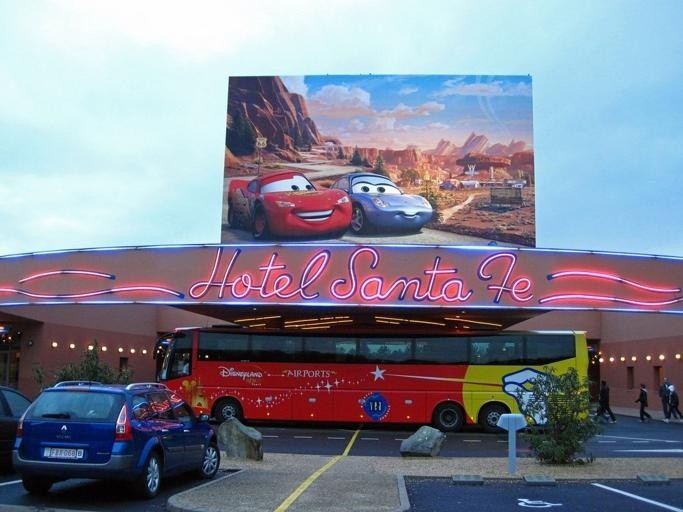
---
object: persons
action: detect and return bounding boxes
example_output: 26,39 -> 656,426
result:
589,379 -> 617,424
633,383 -> 651,422
664,384 -> 682,423
658,377 -> 672,422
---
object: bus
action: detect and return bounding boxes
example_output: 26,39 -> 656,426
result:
149,325 -> 594,433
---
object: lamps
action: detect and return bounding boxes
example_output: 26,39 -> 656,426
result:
591,352 -> 681,365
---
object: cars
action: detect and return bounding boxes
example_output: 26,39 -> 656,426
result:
440,178 -> 461,192
0,383 -> 36,475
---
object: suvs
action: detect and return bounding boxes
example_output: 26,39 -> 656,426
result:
7,374 -> 226,499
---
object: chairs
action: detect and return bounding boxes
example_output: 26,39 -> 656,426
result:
94,395 -> 112,418
